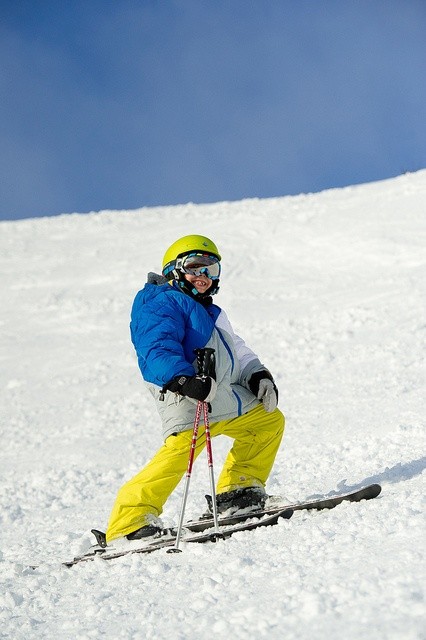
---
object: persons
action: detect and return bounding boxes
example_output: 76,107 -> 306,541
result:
106,235 -> 286,545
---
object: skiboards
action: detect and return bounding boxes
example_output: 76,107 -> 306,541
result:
29,484 -> 382,570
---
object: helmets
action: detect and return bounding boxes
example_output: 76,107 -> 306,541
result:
160,235 -> 223,295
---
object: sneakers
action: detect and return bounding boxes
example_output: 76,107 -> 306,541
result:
204,487 -> 290,517
104,514 -> 180,542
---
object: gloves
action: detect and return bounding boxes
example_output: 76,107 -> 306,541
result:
166,375 -> 217,404
248,373 -> 280,412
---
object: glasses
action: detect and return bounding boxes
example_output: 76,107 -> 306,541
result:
162,252 -> 222,280
